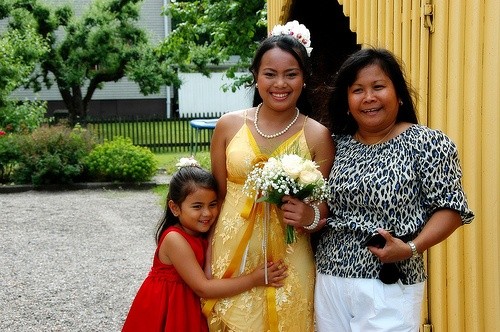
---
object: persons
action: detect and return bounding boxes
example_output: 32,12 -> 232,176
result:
314,49 -> 475,332
121,155 -> 290,332
208,22 -> 334,332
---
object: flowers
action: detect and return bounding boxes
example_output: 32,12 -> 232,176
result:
271,20 -> 313,58
244,150 -> 330,245
176,155 -> 201,168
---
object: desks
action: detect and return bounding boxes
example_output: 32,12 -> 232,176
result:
190,119 -> 218,156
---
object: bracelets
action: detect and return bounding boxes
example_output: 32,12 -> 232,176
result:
303,202 -> 321,230
407,240 -> 420,260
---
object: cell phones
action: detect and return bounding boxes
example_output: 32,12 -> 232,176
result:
363,229 -> 395,247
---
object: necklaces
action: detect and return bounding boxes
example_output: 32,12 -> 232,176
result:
354,131 -> 392,145
252,103 -> 300,140
